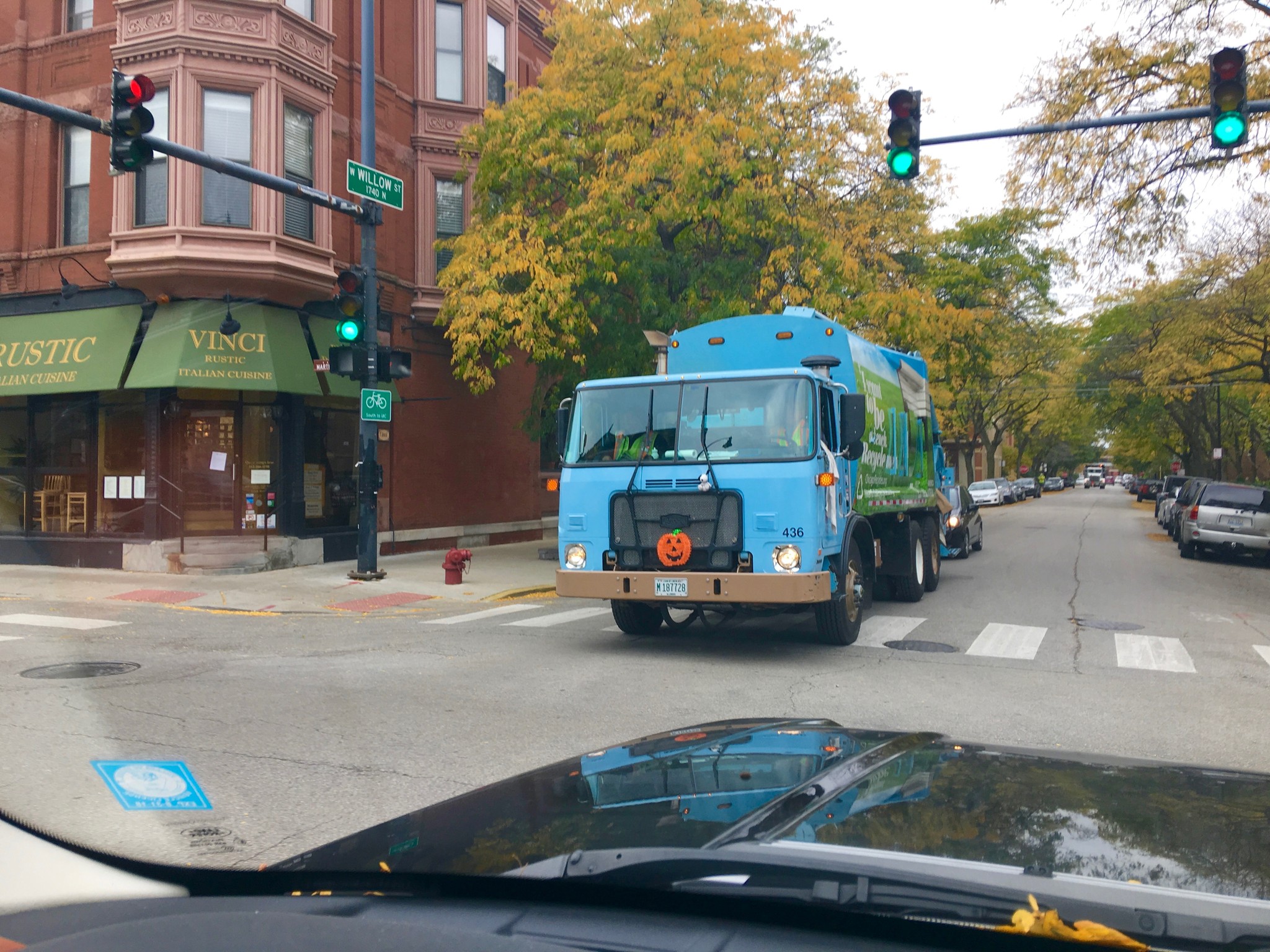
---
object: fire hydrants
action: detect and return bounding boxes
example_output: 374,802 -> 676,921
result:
442,546 -> 473,585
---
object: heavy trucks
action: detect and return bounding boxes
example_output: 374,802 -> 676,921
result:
550,301 -> 957,647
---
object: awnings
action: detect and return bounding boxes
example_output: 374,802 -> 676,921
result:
0,300 -> 402,403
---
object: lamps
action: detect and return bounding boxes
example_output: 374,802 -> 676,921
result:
59,256 -> 117,301
332,294 -> 341,308
219,289 -> 242,335
375,278 -> 381,315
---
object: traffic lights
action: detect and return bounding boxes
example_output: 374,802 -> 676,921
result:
338,269 -> 364,320
109,67 -> 155,175
377,345 -> 412,383
887,90 -> 921,180
328,346 -> 360,377
1209,47 -> 1249,150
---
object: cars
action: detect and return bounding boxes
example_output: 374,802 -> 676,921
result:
1062,477 -> 1075,488
945,483 -> 983,559
1042,477 -> 1065,492
0,714 -> 1269,952
967,480 -> 1005,507
1008,480 -> 1026,501
1018,477 -> 1042,499
984,477 -> 1018,504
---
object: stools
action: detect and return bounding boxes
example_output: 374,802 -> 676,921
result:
23,475 -> 70,532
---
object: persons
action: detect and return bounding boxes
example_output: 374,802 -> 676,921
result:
598,405 -> 671,459
1038,472 -> 1045,491
768,399 -> 827,455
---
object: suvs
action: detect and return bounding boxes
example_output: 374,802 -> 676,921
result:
1105,468 -> 1270,569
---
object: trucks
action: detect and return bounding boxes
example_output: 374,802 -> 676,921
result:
1084,463 -> 1106,489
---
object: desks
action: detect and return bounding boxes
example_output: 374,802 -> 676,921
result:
67,492 -> 87,533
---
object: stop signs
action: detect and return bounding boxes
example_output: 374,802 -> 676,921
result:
1170,461 -> 1181,473
1020,465 -> 1029,474
